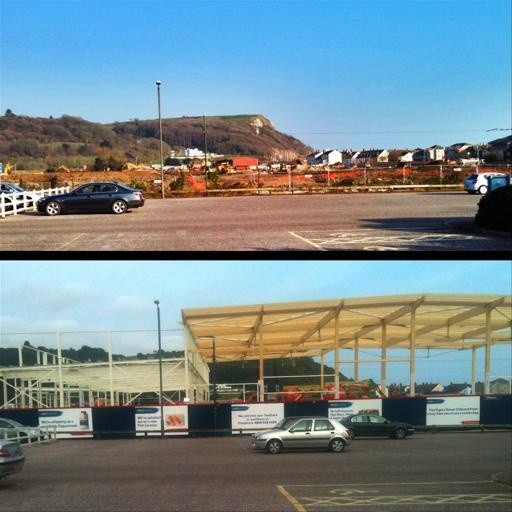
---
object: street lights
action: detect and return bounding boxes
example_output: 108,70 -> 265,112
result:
154,299 -> 165,439
156,79 -> 165,198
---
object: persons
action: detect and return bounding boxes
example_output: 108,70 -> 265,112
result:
275,378 -> 433,399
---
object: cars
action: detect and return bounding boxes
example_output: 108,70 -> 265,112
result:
35,181 -> 145,216
0,181 -> 32,210
0,437 -> 24,480
465,172 -> 506,193
476,184 -> 512,235
252,417 -> 353,454
338,414 -> 417,440
